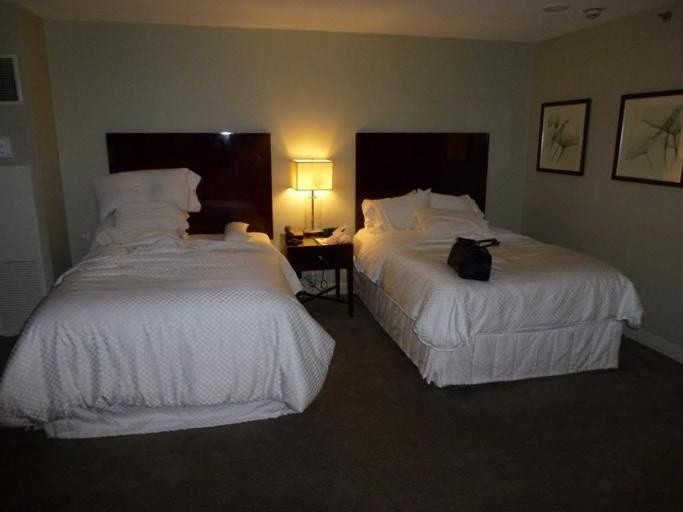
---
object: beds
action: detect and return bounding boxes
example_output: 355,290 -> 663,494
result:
348,129 -> 643,387
2,132 -> 336,438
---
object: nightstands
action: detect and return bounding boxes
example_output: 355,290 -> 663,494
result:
283,230 -> 354,320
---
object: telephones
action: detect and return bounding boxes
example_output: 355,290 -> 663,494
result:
285,225 -> 304,239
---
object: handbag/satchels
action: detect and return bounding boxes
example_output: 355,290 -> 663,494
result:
447,237 -> 501,281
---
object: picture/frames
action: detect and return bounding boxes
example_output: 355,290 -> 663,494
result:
534,97 -> 592,176
608,88 -> 682,189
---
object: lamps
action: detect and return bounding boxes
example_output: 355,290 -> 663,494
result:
287,158 -> 334,236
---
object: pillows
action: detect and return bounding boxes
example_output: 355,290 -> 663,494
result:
92,166 -> 203,241
361,188 -> 490,234
